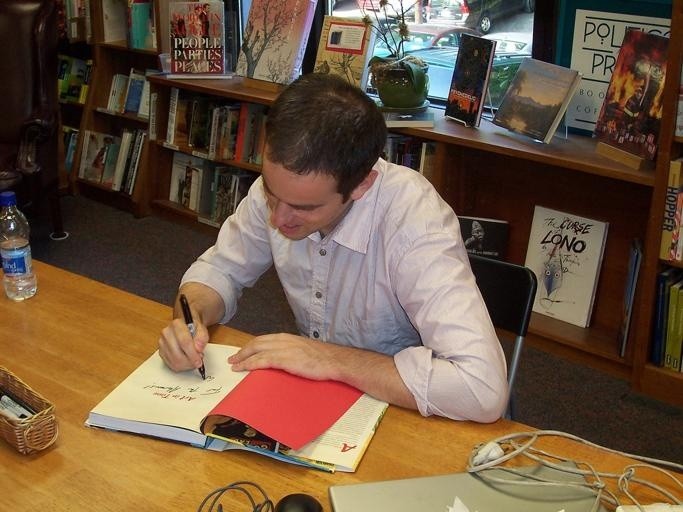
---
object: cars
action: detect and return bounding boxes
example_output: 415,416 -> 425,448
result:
331,11 -> 403,40
479,28 -> 532,55
370,24 -> 484,86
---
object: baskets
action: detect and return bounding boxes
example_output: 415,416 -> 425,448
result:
0,365 -> 58,455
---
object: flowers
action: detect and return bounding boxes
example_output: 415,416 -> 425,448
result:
361,0 -> 429,95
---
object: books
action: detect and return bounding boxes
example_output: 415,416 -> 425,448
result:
53,53 -> 93,104
314,14 -> 379,93
107,69 -> 153,120
236,1 -> 318,85
102,1 -> 158,49
381,134 -> 435,181
457,216 -> 510,264
616,239 -> 641,357
647,158 -> 681,371
55,2 -> 94,45
78,129 -> 149,196
167,151 -> 255,228
84,343 -> 389,475
168,2 -> 227,74
591,28 -> 675,160
166,85 -> 269,165
524,206 -> 610,329
59,125 -> 79,173
443,32 -> 497,128
492,57 -> 584,143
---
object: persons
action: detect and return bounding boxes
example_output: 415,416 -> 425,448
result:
464,220 -> 502,262
157,71 -> 511,426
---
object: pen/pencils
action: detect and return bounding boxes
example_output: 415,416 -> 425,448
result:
0,387 -> 37,418
179,295 -> 205,379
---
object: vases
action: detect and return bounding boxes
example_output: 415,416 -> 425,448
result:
371,63 -> 429,108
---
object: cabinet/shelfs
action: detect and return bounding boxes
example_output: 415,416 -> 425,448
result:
144,72 -> 656,383
57,0 -> 171,219
626,0 -> 682,407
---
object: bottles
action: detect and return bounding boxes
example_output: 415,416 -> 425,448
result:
0,190 -> 36,301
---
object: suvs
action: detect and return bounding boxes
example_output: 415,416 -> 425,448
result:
386,0 -> 533,32
387,45 -> 532,108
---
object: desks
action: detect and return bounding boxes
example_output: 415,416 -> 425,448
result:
0,257 -> 683,512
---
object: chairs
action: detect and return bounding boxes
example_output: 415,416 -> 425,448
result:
467,252 -> 537,420
